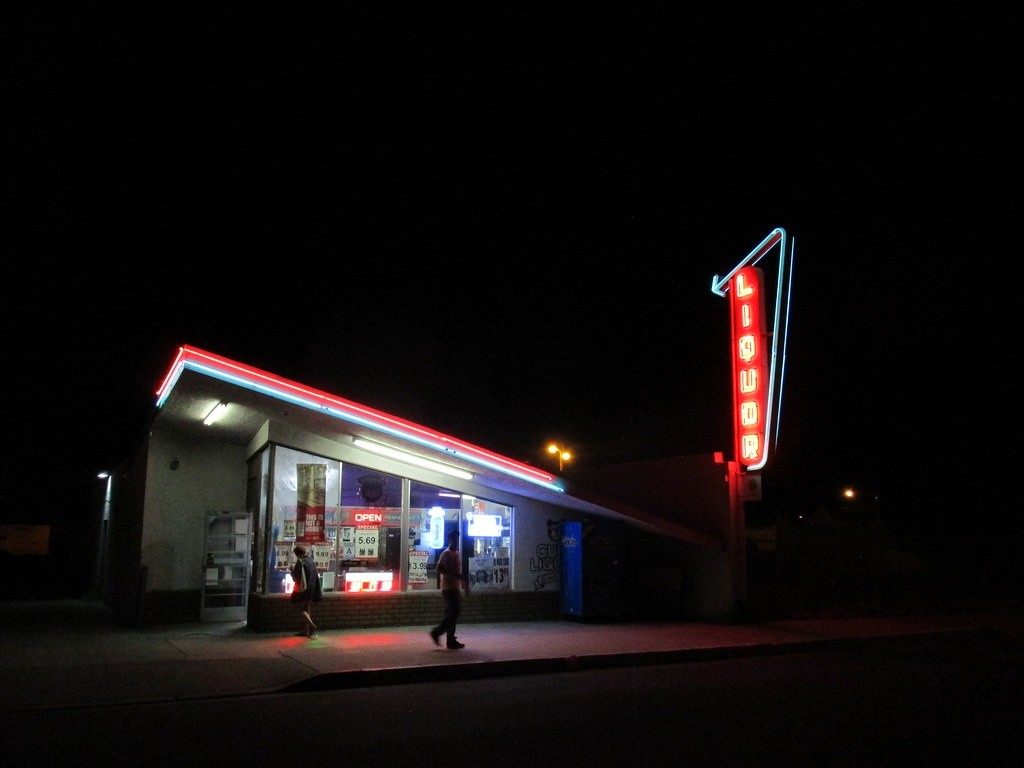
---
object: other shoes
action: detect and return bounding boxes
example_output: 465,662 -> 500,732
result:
430,631 -> 440,645
447,640 -> 465,649
308,624 -> 317,638
294,631 -> 307,636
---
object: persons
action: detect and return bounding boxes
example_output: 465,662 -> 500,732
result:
429,530 -> 466,649
290,547 -> 322,637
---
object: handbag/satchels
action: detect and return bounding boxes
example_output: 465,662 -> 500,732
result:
294,558 -> 308,593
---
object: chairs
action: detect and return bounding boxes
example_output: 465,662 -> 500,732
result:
320,572 -> 334,592
